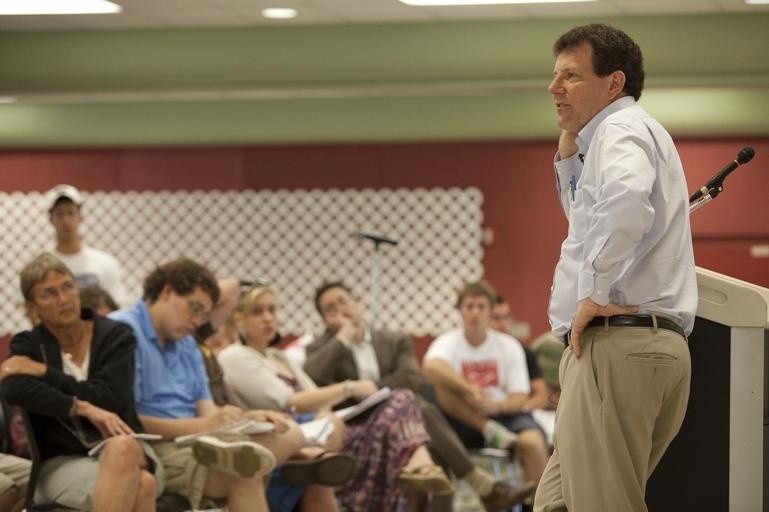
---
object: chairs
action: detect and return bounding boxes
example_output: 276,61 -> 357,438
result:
21,402 -> 66,512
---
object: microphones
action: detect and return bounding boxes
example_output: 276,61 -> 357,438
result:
360,230 -> 398,245
697,147 -> 755,196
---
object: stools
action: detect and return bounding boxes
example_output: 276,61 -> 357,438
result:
470,449 -> 525,512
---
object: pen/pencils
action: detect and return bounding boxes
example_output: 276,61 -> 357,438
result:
222,395 -> 230,404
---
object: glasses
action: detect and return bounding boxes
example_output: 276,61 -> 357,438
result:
239,275 -> 272,292
187,294 -> 209,324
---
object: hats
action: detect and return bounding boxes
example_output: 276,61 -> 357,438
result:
43,184 -> 87,211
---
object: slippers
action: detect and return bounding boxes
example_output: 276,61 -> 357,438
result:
399,465 -> 453,495
267,450 -> 356,487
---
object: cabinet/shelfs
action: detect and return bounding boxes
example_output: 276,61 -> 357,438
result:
644,264 -> 769,512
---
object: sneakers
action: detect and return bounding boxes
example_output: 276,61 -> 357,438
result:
193,436 -> 277,482
483,422 -> 519,450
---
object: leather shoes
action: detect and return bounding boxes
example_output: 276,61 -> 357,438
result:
479,480 -> 537,512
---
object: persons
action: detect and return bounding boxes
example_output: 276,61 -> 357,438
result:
42,185 -> 125,308
532,23 -> 698,511
1,252 -> 560,511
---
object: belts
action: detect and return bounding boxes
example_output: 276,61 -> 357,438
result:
564,315 -> 688,349
